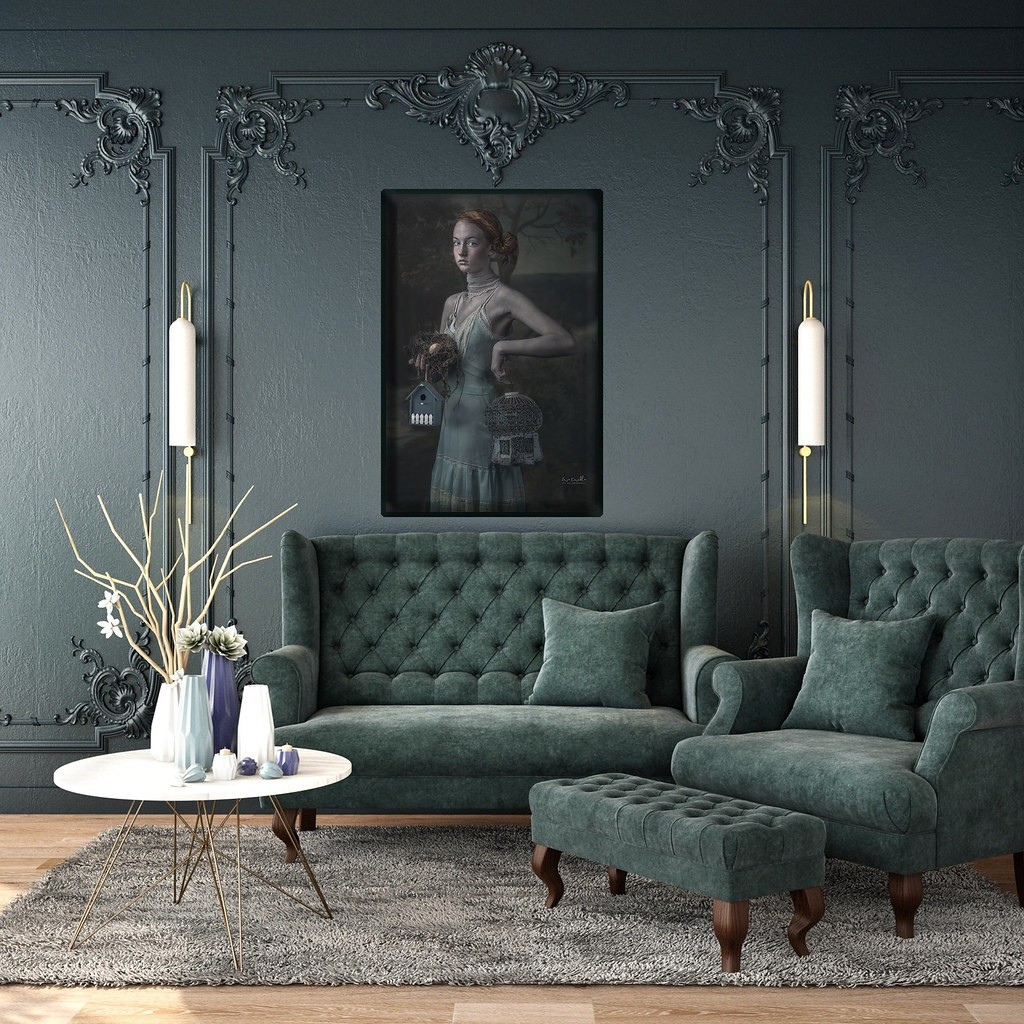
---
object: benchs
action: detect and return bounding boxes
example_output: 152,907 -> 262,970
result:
529,773 -> 826,974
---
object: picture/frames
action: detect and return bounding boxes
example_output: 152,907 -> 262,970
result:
381,189 -> 603,517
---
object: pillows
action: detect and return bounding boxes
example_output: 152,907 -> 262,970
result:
525,598 -> 665,709
781,609 -> 939,740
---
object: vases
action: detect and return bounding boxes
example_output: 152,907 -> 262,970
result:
150,649 -> 275,771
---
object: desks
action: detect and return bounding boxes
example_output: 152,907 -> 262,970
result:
54,749 -> 352,972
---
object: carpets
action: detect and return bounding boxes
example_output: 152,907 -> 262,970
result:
0,825 -> 1024,984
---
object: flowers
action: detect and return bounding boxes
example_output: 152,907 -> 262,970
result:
51,470 -> 298,681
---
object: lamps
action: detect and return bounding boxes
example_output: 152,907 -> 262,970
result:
798,280 -> 826,525
169,281 -> 196,524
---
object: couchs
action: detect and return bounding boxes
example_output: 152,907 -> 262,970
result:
250,529 -> 740,862
671,532 -> 1024,939
